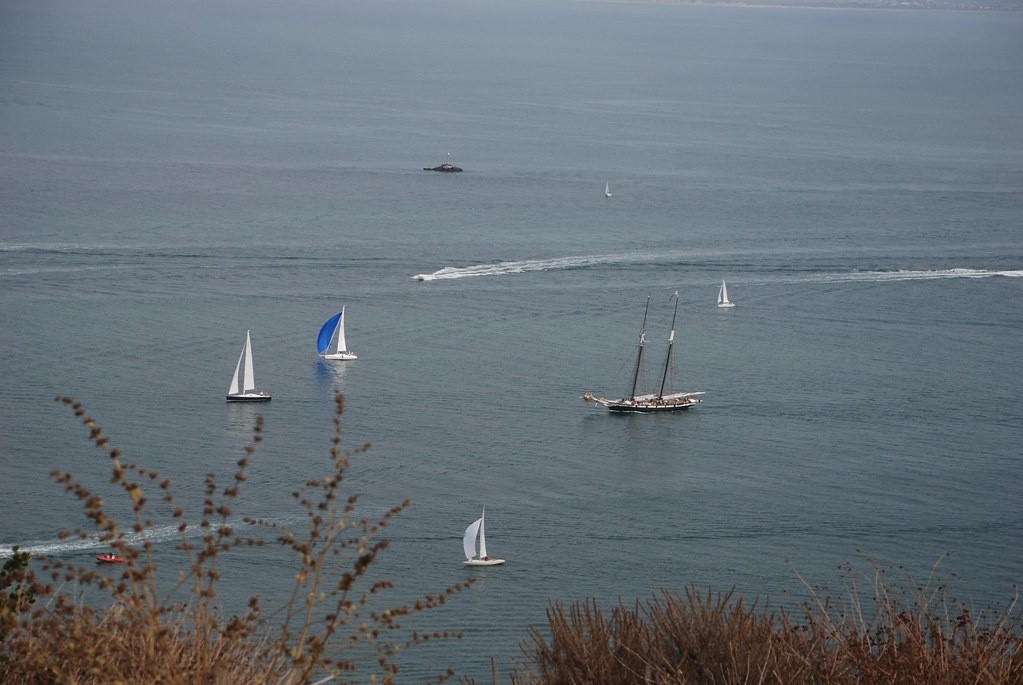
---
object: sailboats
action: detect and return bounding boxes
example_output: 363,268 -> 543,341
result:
716,279 -> 736,308
314,305 -> 359,360
582,291 -> 707,414
463,504 -> 506,566
224,329 -> 272,402
